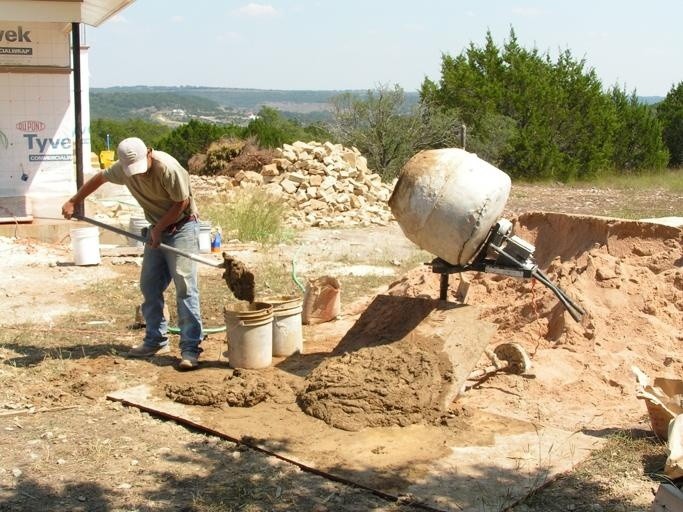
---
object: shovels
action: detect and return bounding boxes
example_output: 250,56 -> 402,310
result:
73,213 -> 255,304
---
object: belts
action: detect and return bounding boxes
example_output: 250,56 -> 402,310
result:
167,214 -> 199,232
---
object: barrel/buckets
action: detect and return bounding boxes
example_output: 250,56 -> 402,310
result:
223,302 -> 273,370
258,293 -> 303,357
198,223 -> 212,255
129,217 -> 150,247
69,225 -> 101,267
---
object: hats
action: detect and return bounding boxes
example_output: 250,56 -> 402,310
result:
117,137 -> 148,176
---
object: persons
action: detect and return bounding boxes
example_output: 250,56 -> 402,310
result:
61,137 -> 206,369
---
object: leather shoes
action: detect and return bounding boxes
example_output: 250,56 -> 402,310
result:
179,351 -> 198,369
129,346 -> 169,356
